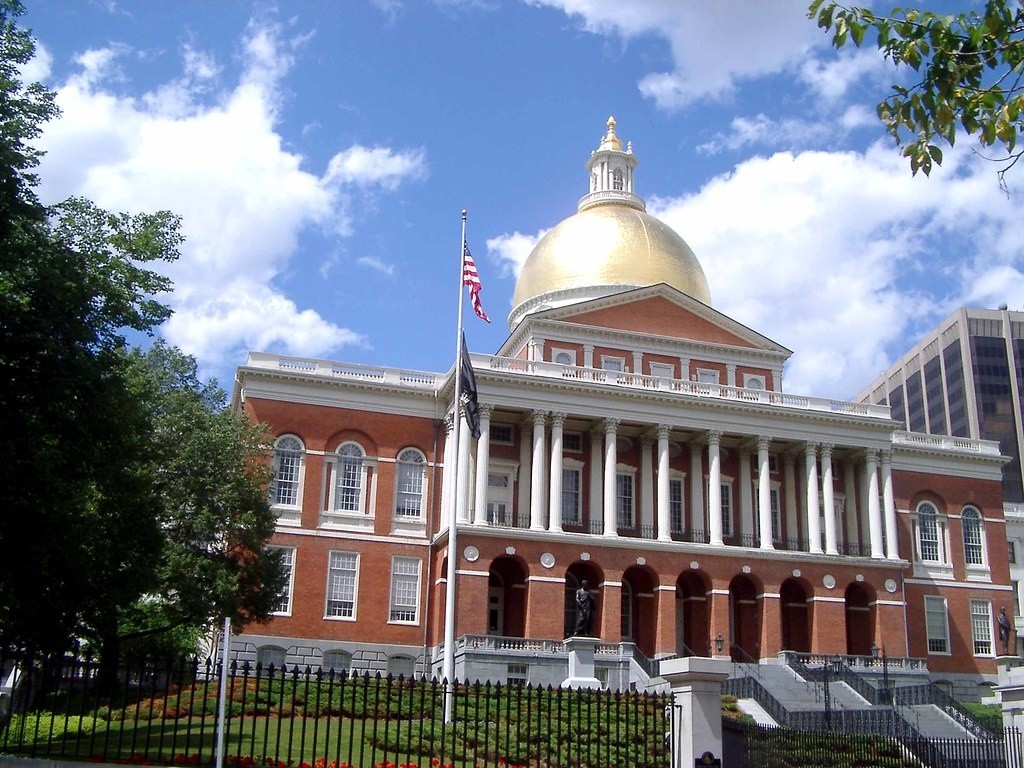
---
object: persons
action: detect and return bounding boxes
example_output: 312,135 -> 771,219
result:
573,579 -> 594,636
996,606 -> 1017,655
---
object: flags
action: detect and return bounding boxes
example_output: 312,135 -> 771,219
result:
463,240 -> 491,323
461,328 -> 481,440
